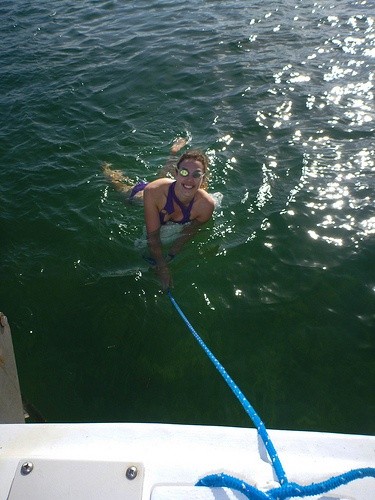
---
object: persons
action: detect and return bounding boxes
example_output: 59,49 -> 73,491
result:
100,136 -> 217,291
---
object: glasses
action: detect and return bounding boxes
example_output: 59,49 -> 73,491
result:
176,168 -> 203,178
